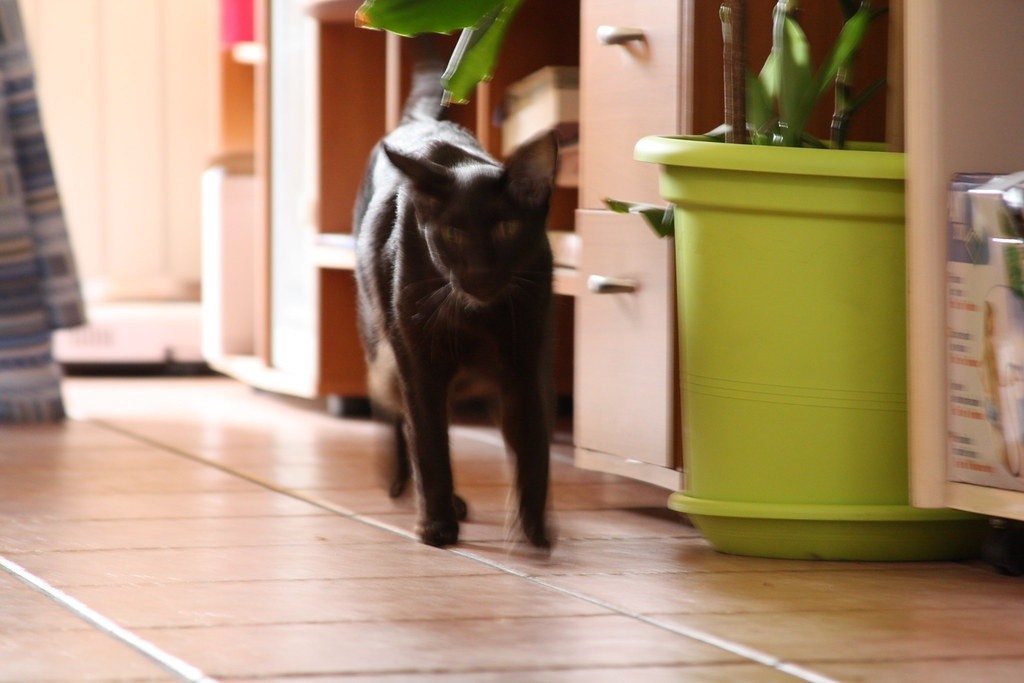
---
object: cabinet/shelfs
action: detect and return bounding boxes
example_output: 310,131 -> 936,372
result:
203,0 -> 890,492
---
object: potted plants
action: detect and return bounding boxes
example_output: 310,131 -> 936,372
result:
606,0 -> 979,561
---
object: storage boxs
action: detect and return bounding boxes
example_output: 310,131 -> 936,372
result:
946,172 -> 1024,492
500,67 -> 580,159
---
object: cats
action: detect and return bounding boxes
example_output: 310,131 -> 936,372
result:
353,33 -> 559,557
